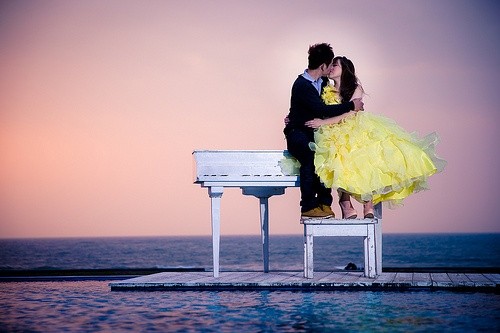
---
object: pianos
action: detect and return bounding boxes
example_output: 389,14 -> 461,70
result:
190,149 -> 386,278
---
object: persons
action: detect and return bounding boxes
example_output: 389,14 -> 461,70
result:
282,43 -> 365,219
284,54 -> 448,219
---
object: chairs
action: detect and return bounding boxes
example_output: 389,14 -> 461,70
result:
302,186 -> 382,280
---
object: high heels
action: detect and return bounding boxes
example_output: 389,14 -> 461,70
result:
362,204 -> 374,218
339,201 -> 358,219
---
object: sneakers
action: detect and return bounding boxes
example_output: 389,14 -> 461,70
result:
319,204 -> 335,219
301,207 -> 334,220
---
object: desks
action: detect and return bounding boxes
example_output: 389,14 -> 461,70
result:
192,148 -> 302,279
